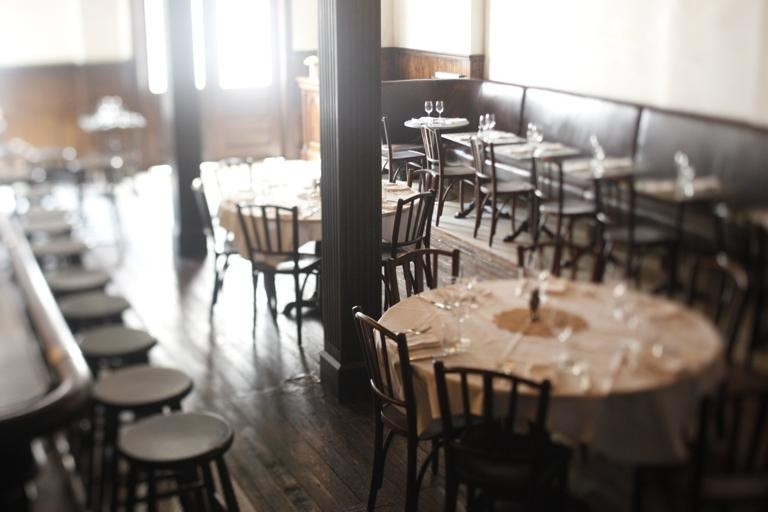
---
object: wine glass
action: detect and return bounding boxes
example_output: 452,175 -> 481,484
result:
423,100 -> 444,125
477,113 -> 496,141
547,306 -> 573,374
587,135 -> 606,179
526,122 -> 544,155
442,274 -> 473,354
675,153 -> 696,201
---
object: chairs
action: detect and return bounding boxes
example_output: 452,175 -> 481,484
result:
192,177 -> 239,318
236,205 -> 322,344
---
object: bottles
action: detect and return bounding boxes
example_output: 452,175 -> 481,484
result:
529,289 -> 542,324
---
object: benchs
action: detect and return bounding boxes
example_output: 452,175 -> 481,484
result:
381,76 -> 768,273
